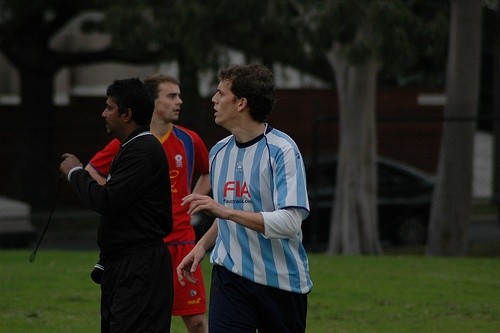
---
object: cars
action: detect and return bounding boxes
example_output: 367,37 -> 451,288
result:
303,153 -> 435,252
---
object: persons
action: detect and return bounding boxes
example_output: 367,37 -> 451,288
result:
85,74 -> 206,332
175,62 -> 313,333
59,76 -> 174,332
